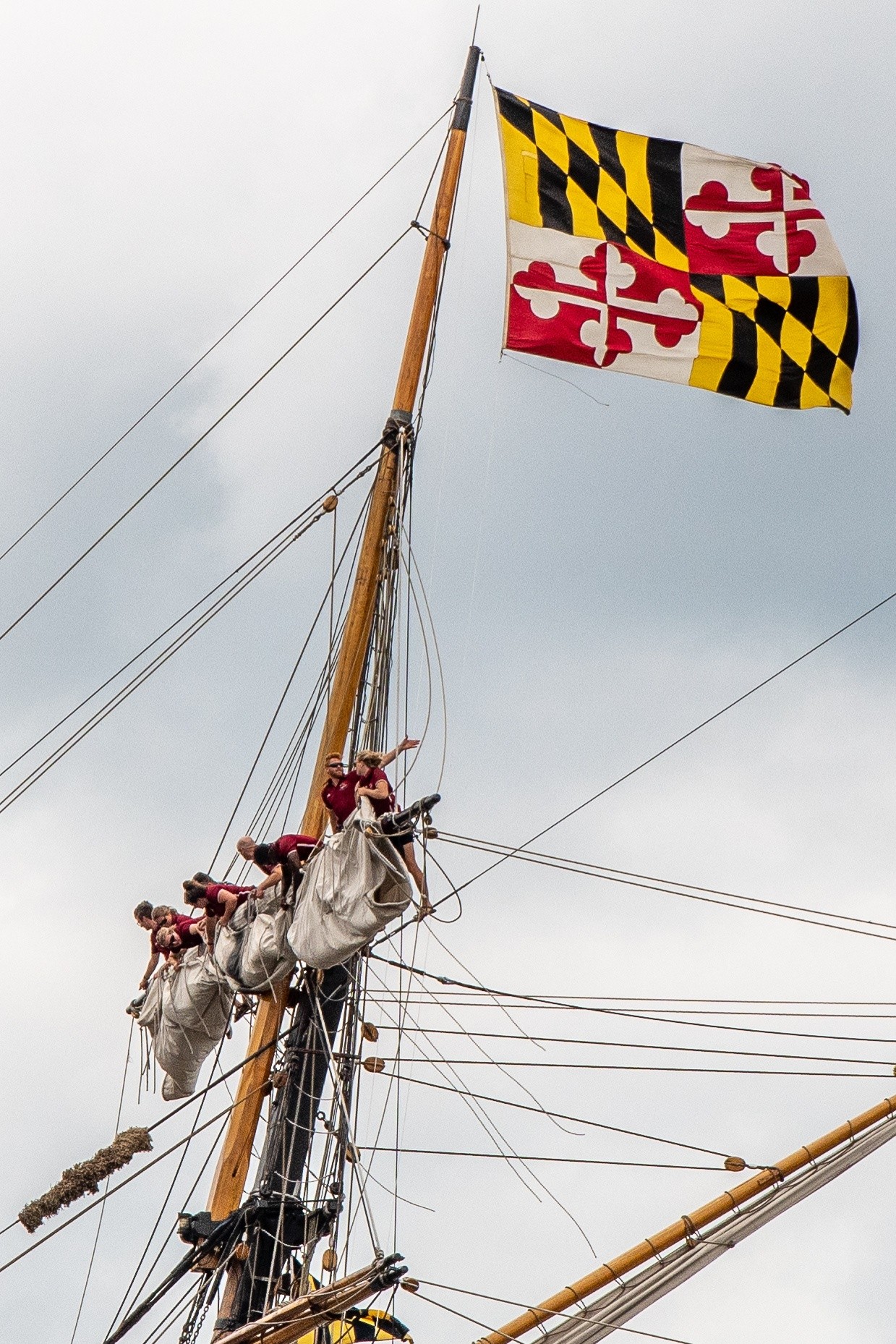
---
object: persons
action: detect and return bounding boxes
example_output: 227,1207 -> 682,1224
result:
237,834 -> 325,911
133,872 -> 258,991
321,735 -> 433,922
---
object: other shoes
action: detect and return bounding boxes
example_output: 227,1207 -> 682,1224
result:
227,1025 -> 233,1039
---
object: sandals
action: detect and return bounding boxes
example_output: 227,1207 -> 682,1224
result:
413,902 -> 437,923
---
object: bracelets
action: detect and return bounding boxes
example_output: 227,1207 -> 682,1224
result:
207,941 -> 214,946
143,976 -> 150,980
357,788 -> 365,796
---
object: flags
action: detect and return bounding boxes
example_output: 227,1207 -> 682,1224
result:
492,86 -> 859,419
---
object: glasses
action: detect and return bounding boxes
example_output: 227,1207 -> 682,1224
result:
353,762 -> 358,768
167,932 -> 175,948
137,919 -> 142,926
159,915 -> 167,926
328,762 -> 344,768
